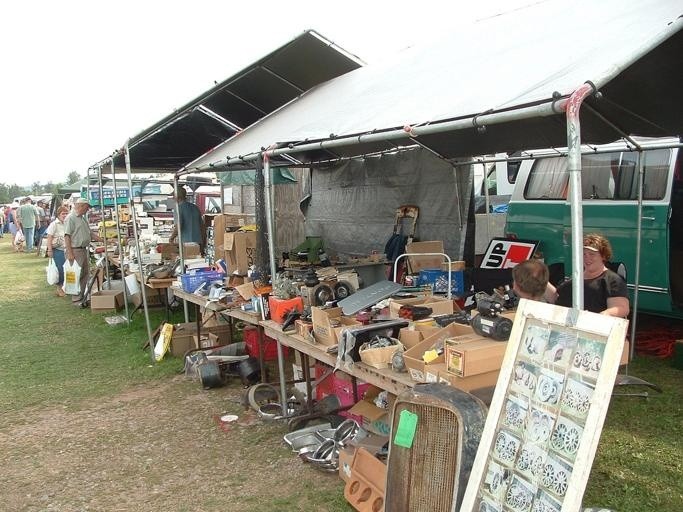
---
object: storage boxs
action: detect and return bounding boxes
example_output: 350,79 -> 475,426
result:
171,320 -> 233,357
242,327 -> 289,361
310,295 -> 510,422
90,290 -> 123,313
183,242 -> 200,258
224,231 -> 256,278
405,241 -> 444,273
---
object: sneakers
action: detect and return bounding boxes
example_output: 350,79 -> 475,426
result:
74,300 -> 82,305
57,289 -> 67,297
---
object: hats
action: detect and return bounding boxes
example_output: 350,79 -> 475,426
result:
583,245 -> 598,252
170,189 -> 186,196
77,198 -> 92,206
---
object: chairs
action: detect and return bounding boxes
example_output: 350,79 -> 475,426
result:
608,260 -> 662,402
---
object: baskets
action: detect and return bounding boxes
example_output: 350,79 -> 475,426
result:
180,271 -> 223,294
418,269 -> 464,294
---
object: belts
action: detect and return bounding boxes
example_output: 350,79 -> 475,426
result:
71,246 -> 86,249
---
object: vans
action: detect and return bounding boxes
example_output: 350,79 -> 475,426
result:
504,138 -> 683,317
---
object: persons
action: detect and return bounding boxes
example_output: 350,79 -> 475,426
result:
542,233 -> 630,317
64,198 -> 114,309
46,206 -> 70,297
169,186 -> 205,254
511,259 -> 549,301
0,196 -> 50,251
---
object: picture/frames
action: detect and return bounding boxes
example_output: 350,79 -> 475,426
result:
457,298 -> 629,512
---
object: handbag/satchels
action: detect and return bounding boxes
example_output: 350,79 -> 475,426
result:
41,235 -> 62,249
